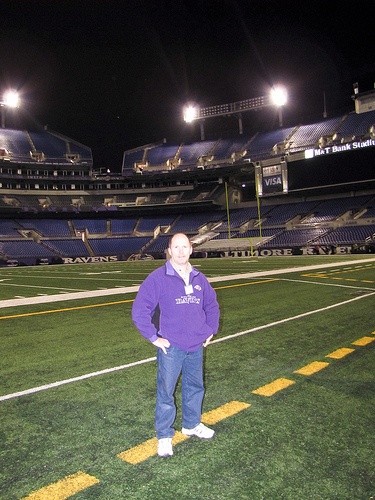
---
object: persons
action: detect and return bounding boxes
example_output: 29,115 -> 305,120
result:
131,233 -> 220,457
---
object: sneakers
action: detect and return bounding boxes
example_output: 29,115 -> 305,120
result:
157,438 -> 173,457
182,423 -> 215,438
